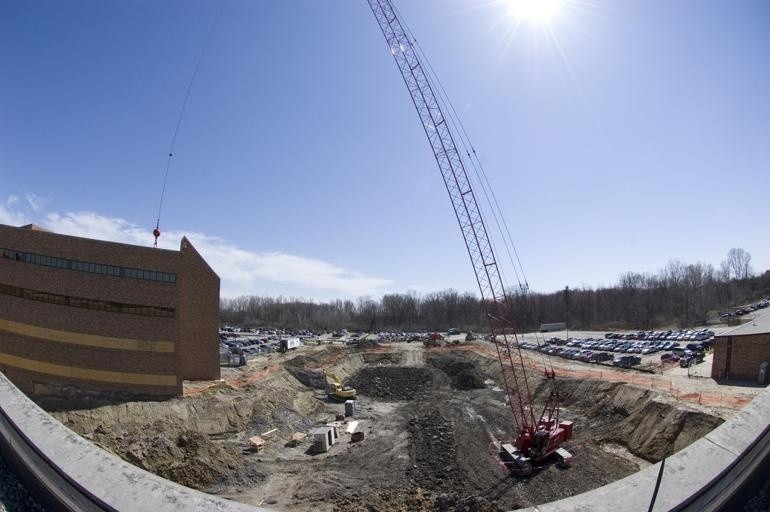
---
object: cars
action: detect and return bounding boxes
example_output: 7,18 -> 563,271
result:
377,327 -> 461,347
484,328 -> 716,368
719,296 -> 770,321
219,323 -> 363,349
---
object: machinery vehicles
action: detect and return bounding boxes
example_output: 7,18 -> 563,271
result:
321,368 -> 357,403
364,1 -> 576,478
